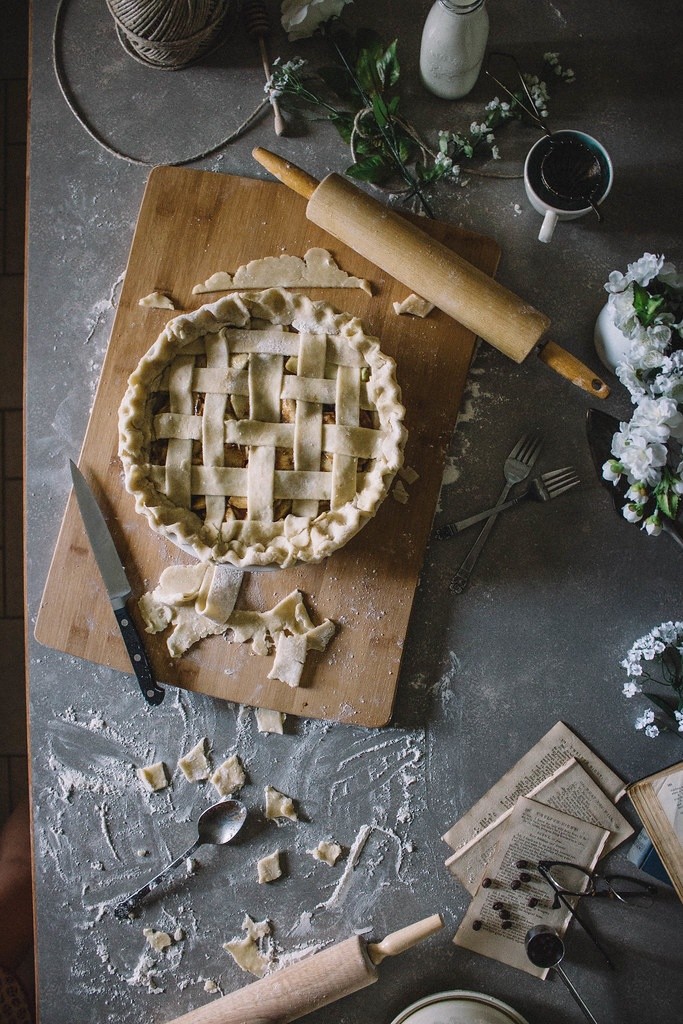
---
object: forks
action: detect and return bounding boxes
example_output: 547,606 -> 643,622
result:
449,430 -> 547,597
433,464 -> 580,541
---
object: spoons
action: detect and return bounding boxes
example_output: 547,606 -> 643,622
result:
114,800 -> 247,921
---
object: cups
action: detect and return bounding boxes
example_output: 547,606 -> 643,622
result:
524,130 -> 614,244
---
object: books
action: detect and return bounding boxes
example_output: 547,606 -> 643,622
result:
627,760 -> 682,903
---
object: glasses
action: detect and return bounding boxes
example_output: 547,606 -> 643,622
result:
538,861 -> 656,964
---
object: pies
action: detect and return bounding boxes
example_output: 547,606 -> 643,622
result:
119,288 -> 406,567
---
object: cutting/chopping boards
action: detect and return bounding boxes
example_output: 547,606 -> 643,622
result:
34,167 -> 500,728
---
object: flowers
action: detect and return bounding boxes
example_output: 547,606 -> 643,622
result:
263,0 -> 578,222
617,621 -> 683,740
603,250 -> 683,535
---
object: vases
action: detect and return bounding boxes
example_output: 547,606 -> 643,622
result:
593,302 -> 654,380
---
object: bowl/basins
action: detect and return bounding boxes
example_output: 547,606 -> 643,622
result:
389,989 -> 529,1024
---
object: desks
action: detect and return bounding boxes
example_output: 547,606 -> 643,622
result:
28,0 -> 682,1024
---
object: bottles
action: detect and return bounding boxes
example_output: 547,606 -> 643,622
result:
420,0 -> 490,100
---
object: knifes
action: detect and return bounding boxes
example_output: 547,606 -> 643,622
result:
69,458 -> 165,707
